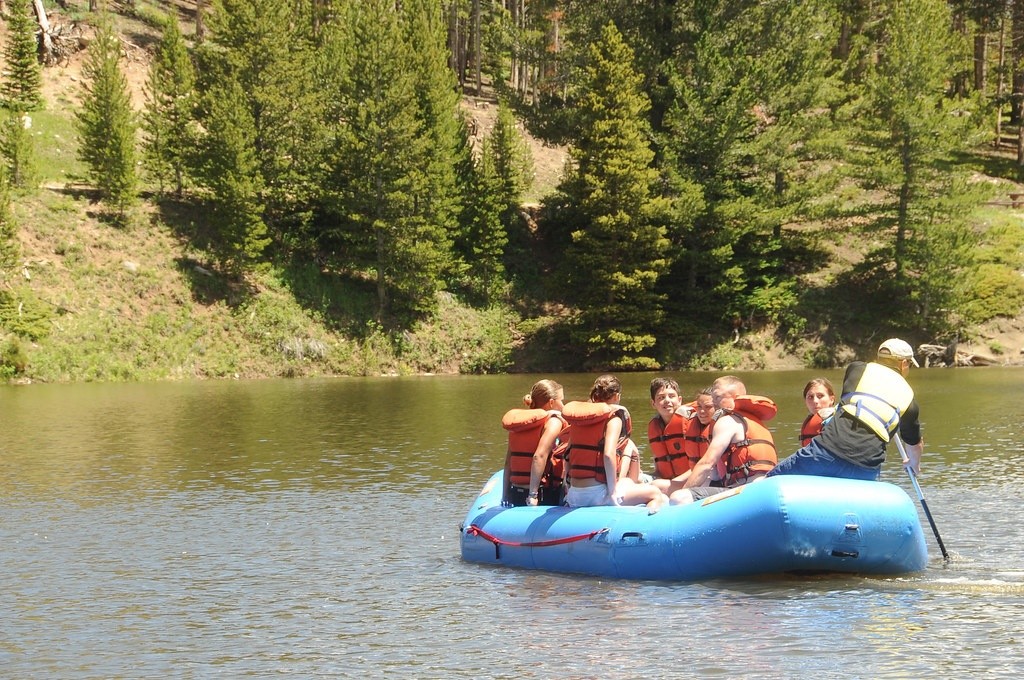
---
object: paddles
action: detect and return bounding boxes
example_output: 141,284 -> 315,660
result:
892,433 -> 949,561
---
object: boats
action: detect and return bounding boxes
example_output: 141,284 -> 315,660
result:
458,468 -> 928,581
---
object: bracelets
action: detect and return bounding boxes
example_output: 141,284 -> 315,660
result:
529,492 -> 538,497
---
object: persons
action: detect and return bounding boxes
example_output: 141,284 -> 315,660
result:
798,378 -> 836,448
764,338 -> 923,480
501,374 -> 663,507
648,375 -> 776,504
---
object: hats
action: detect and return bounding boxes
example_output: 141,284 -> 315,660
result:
877,338 -> 919,368
